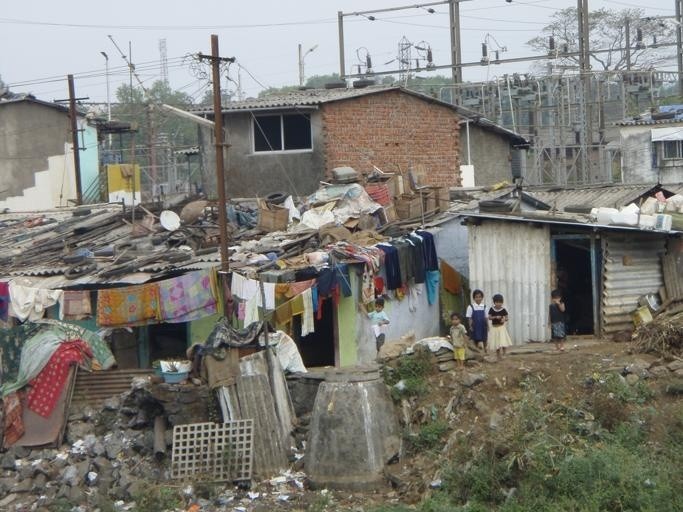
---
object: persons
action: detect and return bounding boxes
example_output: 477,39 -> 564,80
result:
450,290 -> 513,370
548,289 -> 566,350
358,299 -> 390,354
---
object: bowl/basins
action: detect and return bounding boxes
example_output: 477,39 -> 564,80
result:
161,371 -> 190,384
